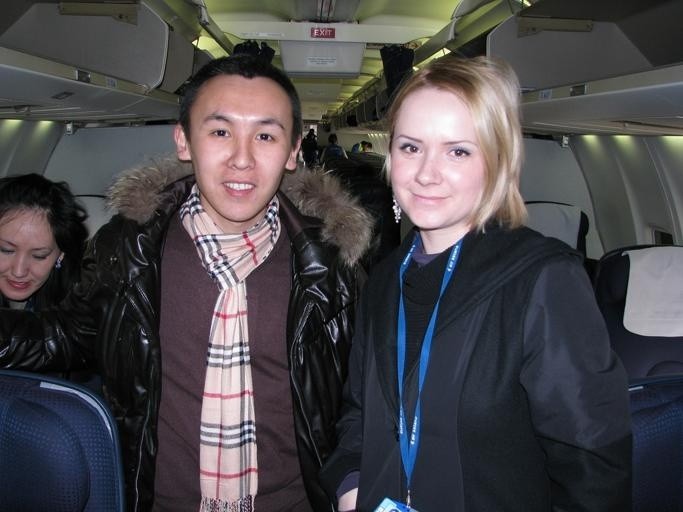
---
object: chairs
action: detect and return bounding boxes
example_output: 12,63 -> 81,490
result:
0,368 -> 125,511
520,201 -> 590,259
594,243 -> 682,510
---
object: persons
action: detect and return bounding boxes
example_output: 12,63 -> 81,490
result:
0,173 -> 102,399
301,129 -> 373,172
315,56 -> 635,512
0,56 -> 379,512
